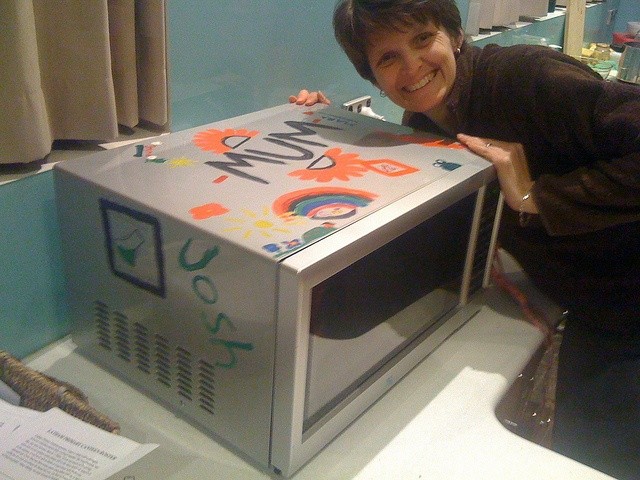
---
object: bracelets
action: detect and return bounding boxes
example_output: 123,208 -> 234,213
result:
516,191 -> 533,228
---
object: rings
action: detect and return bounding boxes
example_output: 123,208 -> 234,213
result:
485,143 -> 492,149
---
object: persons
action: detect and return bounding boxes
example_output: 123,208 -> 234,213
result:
288,0 -> 639,359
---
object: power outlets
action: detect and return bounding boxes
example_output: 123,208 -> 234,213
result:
343,96 -> 371,114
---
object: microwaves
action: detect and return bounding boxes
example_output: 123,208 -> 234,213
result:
53,102 -> 504,476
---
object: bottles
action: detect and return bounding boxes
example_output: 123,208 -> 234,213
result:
593,43 -> 611,60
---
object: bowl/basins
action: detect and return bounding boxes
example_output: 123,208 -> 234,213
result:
627,21 -> 640,38
573,57 -> 613,80
